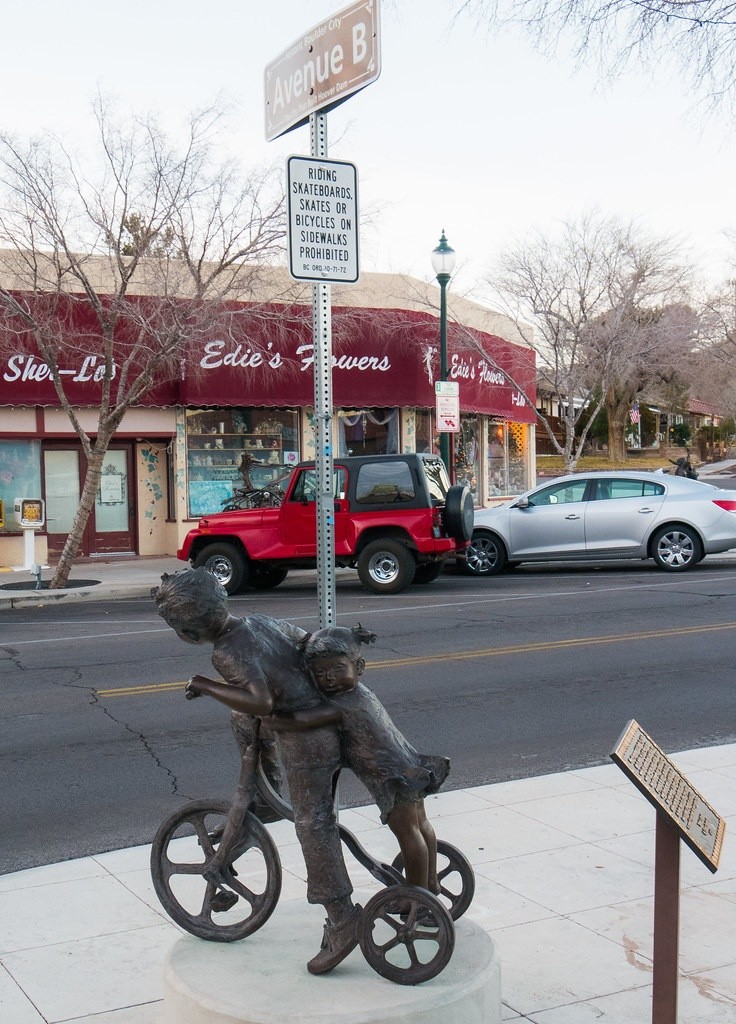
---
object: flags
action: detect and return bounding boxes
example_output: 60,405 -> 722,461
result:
629,404 -> 640,424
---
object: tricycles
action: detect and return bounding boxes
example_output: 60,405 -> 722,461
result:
149,710 -> 477,989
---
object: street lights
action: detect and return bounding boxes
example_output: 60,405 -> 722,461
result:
432,232 -> 457,470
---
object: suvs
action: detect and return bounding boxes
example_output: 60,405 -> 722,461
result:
179,454 -> 477,593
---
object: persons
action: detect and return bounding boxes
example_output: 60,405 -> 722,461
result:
265,621 -> 451,910
148,566 -> 378,972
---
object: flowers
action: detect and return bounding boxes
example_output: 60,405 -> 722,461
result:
456,443 -> 478,492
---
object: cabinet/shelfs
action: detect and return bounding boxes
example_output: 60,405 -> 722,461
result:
187,433 -> 282,482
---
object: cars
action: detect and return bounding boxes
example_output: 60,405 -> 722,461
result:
456,469 -> 735,575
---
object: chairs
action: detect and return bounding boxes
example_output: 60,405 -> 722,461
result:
597,485 -> 611,500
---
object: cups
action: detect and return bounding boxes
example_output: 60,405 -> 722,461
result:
273,469 -> 278,480
204,442 -> 211,449
340,492 -> 345,499
243,439 -> 251,448
264,475 -> 272,480
218,421 -> 225,434
